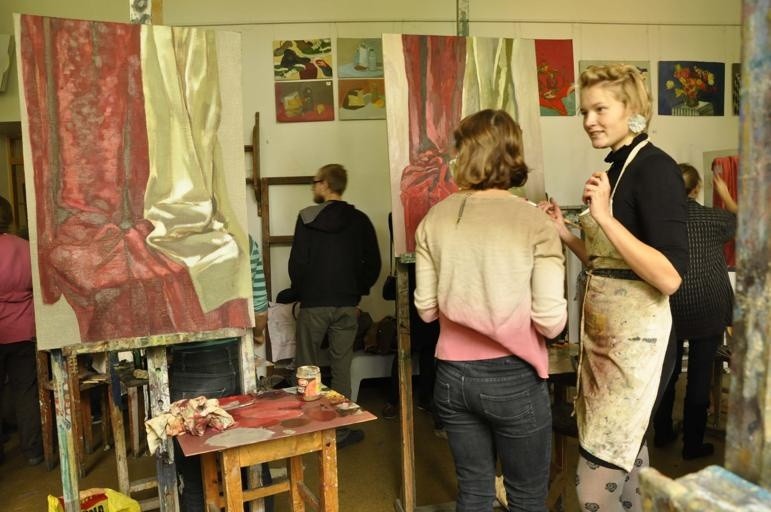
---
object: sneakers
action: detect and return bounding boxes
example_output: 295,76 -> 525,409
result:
433,429 -> 449,440
382,403 -> 398,420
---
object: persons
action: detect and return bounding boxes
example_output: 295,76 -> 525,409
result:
652,163 -> 736,461
166,234 -> 275,511
410,107 -> 567,512
0,195 -> 44,467
286,163 -> 383,452
536,63 -> 690,512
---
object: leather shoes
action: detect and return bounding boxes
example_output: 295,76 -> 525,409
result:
681,442 -> 714,460
337,429 -> 364,449
653,431 -> 679,447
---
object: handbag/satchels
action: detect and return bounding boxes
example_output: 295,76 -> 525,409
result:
382,213 -> 397,301
372,315 -> 398,357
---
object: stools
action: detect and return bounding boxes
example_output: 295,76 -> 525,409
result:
199,428 -> 340,511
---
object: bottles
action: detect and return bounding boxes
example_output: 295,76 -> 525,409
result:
296,365 -> 323,404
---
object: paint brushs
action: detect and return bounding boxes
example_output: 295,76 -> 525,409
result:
526,199 -> 583,230
